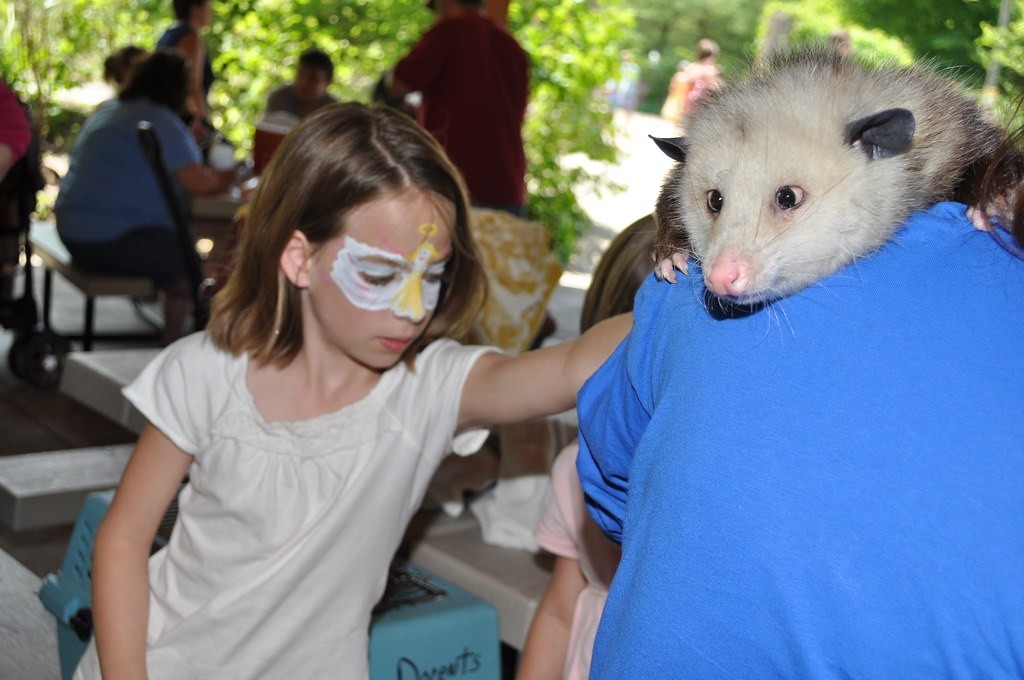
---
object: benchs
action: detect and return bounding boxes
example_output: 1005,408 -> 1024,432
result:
0,444 -> 137,531
0,548 -> 64,680
60,348 -> 556,651
25,222 -> 158,352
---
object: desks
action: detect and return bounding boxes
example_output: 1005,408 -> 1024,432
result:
189,186 -> 247,266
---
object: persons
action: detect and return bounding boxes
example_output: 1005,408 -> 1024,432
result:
831,30 -> 852,56
74,102 -> 633,679
55,0 -> 250,347
679,40 -> 719,125
254,49 -> 339,177
371,0 -> 557,351
577,125 -> 1024,680
0,77 -> 41,297
517,213 -> 658,680
